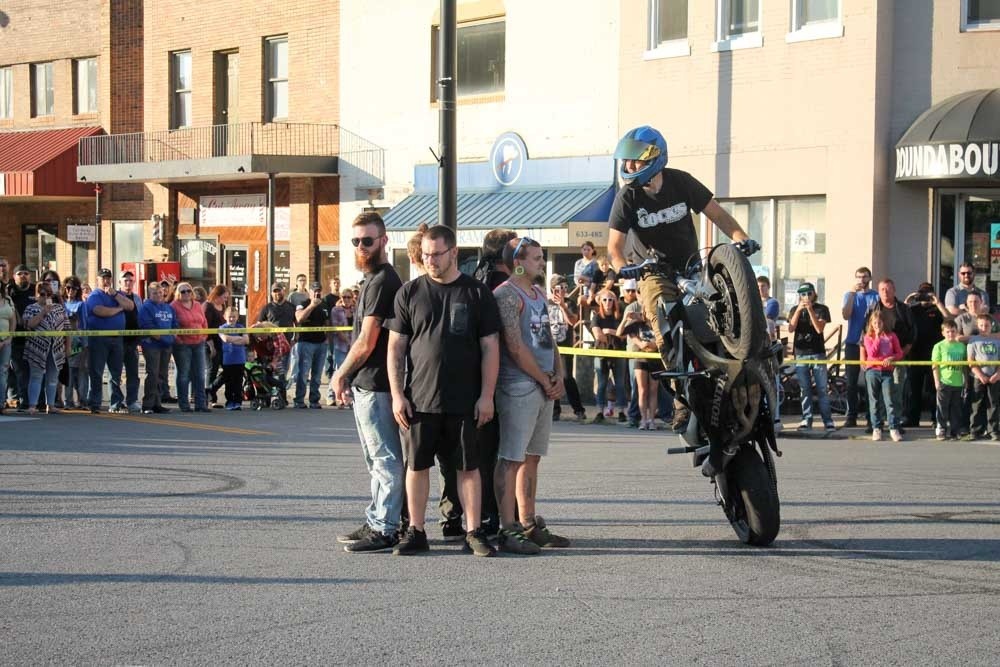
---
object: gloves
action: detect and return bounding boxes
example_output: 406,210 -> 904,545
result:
619,264 -> 641,281
738,240 -> 761,256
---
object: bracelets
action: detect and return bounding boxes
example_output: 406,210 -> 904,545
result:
113,292 -> 118,297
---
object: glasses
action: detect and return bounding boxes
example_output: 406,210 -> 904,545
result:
40,293 -> 51,296
342,296 -> 352,299
65,286 -> 79,290
44,278 -> 59,282
603,298 -> 613,302
16,272 -> 28,275
352,234 -> 384,246
960,272 -> 974,275
625,289 -> 636,293
514,237 -> 531,258
801,292 -> 812,297
556,285 -> 568,290
181,289 -> 191,293
422,247 -> 454,261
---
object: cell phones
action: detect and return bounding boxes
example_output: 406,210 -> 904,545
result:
50,281 -> 58,295
916,294 -> 931,301
47,299 -> 53,305
554,285 -> 561,294
630,312 -> 641,319
317,294 -> 320,299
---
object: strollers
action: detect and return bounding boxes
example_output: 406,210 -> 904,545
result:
243,365 -> 291,410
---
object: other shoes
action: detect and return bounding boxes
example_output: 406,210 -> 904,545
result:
552,408 -> 670,430
0,397 -> 337,415
798,420 -> 1000,442
672,406 -> 689,432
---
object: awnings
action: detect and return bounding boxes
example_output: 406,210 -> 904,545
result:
894,88 -> 1000,185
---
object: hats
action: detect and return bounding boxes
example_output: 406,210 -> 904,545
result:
273,283 -> 282,291
98,269 -> 111,277
120,271 -> 133,277
798,283 -> 814,293
310,282 -> 322,290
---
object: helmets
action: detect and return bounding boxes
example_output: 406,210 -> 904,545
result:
614,126 -> 668,190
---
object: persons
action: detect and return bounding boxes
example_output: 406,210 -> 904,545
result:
531,242 -> 1000,442
0,258 -> 368,415
330,212 -> 568,556
607,125 -> 762,434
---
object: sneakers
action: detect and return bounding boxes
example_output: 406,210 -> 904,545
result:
338,515 -> 571,557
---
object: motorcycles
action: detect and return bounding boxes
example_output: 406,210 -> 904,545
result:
613,242 -> 787,550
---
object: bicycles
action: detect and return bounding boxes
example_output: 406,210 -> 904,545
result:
777,365 -> 865,414
